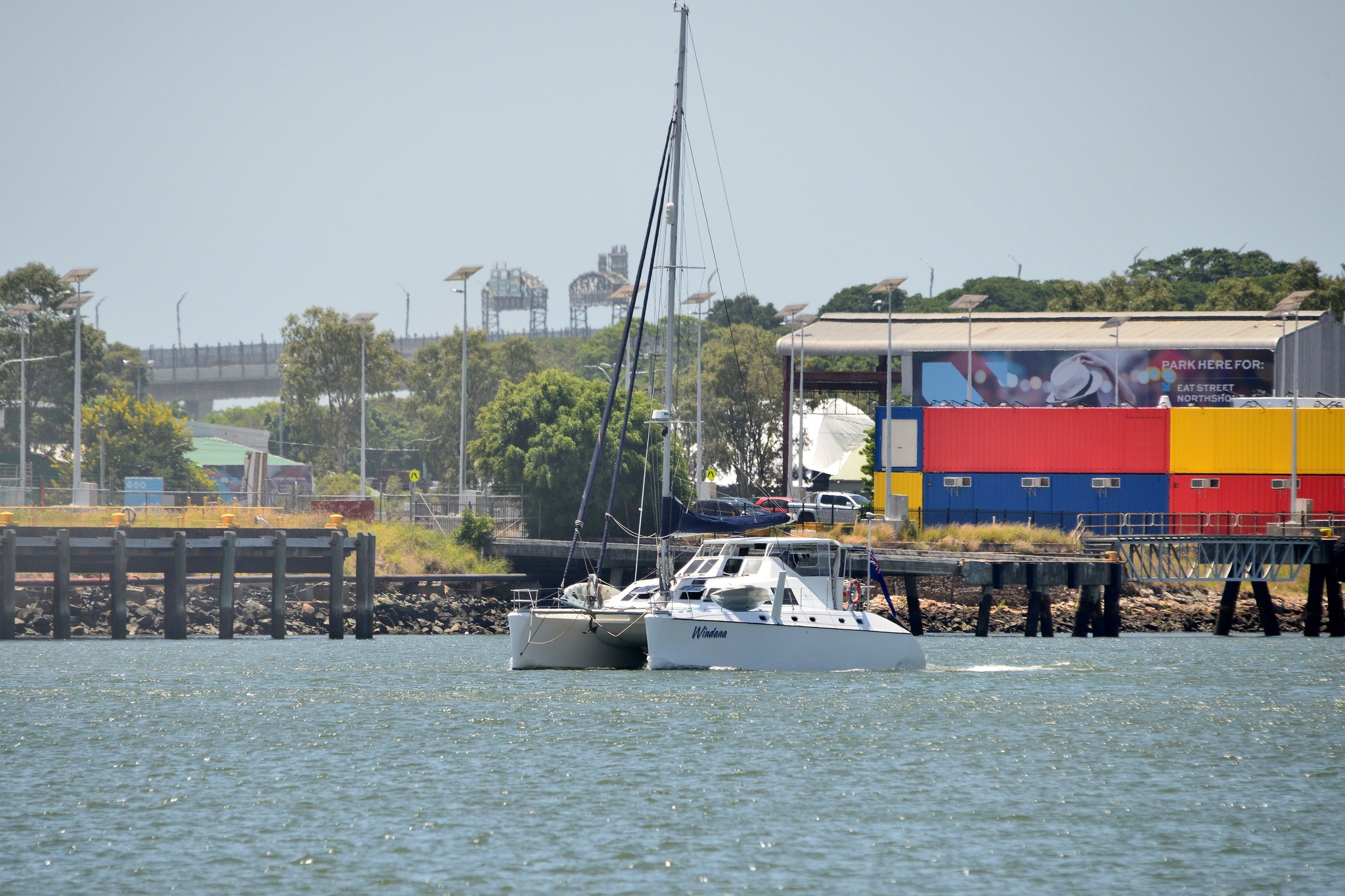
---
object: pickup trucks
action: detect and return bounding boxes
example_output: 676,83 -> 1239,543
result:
788,492 -> 874,523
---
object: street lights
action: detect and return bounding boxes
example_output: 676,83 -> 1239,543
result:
94,295 -> 108,331
441,264 -> 486,514
176,290 -> 190,365
610,284 -> 646,392
772,303 -> 808,495
394,281 -> 411,340
348,311 -> 380,499
779,313 -> 817,499
1262,289 -> 1316,525
5,300 -> 41,505
946,294 -> 989,404
679,290 -> 715,495
1098,315 -> 1135,406
57,265 -> 99,508
867,275 -> 909,517
122,359 -> 156,421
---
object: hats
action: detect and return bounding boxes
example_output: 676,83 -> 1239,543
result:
1046,357 -> 1103,407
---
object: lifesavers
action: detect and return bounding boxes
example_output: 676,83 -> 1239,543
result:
846,579 -> 862,604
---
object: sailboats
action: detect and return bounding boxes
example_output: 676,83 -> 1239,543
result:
505,0 -> 929,671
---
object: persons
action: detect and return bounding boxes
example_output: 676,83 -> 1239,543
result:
1045,352 -> 1136,407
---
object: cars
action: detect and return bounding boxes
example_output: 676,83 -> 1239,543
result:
712,497 -> 772,514
692,499 -> 765,517
755,497 -> 802,512
